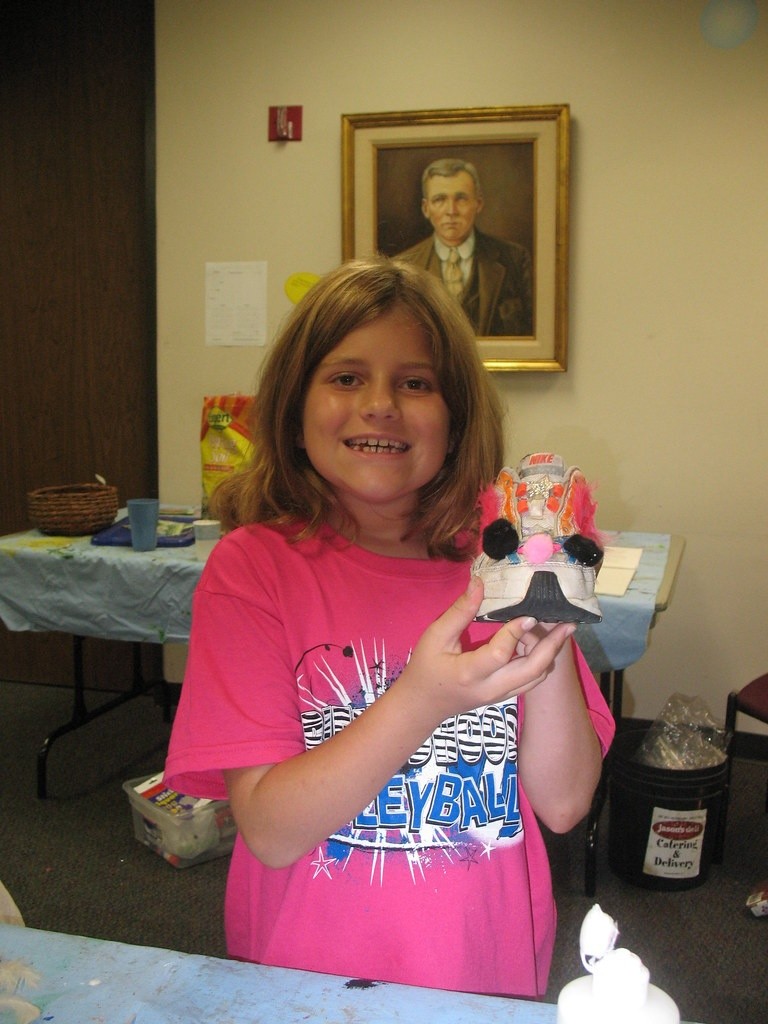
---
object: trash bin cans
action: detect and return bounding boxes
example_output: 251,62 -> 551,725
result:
607,729 -> 729,892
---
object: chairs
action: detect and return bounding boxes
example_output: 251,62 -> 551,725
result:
720,668 -> 768,813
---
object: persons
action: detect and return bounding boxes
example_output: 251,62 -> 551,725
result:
166,258 -> 614,1002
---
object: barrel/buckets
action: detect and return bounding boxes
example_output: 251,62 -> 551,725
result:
608,729 -> 732,893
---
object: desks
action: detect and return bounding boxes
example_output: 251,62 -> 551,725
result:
0,506 -> 684,903
0,926 -> 559,1024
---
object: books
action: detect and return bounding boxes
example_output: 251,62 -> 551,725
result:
135,763 -> 236,867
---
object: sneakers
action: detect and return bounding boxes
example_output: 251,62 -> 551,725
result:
469,454 -> 605,623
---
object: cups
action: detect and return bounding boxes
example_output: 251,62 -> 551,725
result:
127,499 -> 159,552
192,520 -> 221,562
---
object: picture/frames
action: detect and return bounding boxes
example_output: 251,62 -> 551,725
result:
339,103 -> 572,374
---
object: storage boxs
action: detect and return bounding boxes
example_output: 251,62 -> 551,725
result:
121,773 -> 238,869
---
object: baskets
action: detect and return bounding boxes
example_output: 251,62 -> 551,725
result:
26,483 -> 118,535
